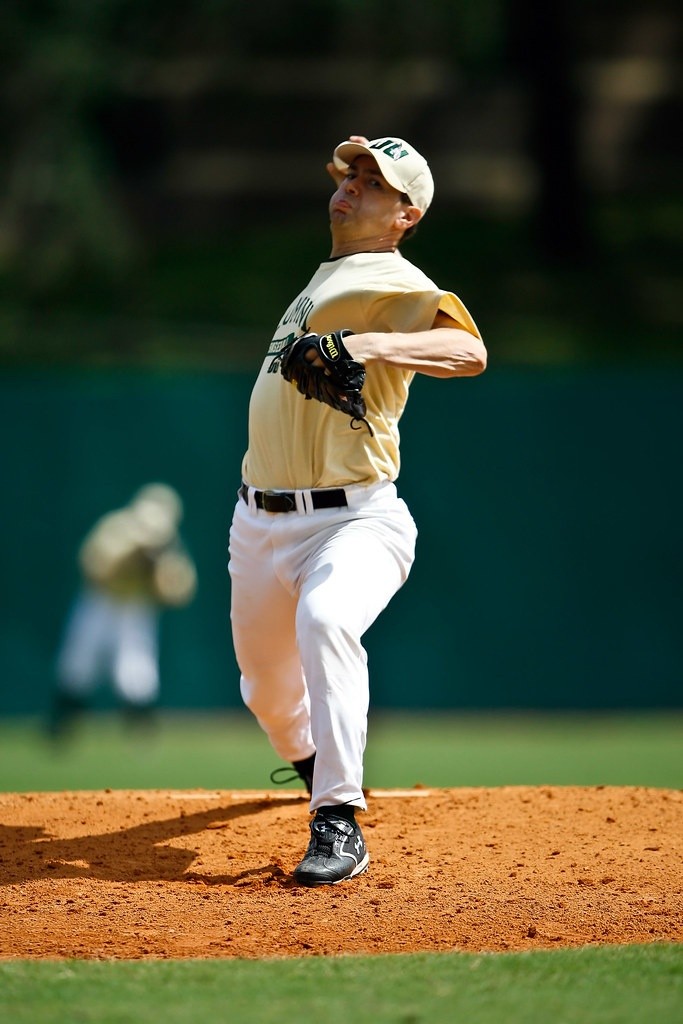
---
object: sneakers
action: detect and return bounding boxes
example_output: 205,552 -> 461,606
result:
293,810 -> 370,885
270,758 -> 313,801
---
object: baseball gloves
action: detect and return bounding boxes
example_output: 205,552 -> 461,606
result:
278,325 -> 377,439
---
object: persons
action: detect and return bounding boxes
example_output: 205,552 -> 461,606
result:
45,479 -> 199,753
226,133 -> 489,885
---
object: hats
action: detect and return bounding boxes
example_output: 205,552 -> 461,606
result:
335,137 -> 434,218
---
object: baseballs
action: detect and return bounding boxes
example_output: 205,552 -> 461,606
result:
332,140 -> 358,176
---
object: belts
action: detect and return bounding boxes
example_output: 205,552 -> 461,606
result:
240,481 -> 348,513
